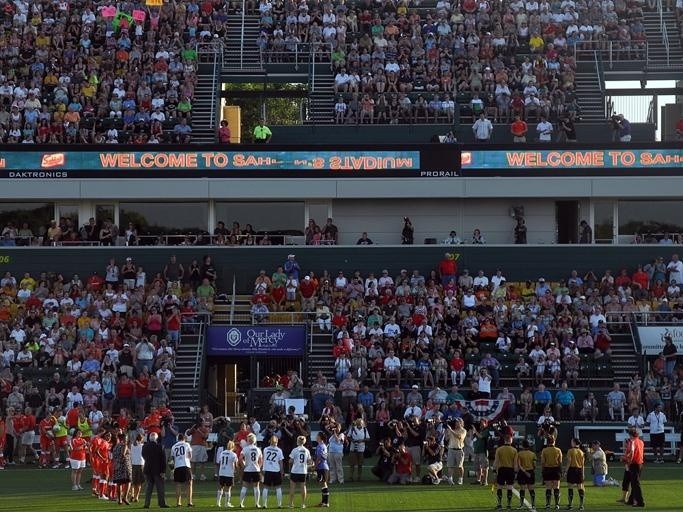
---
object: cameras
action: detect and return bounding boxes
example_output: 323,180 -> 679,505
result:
329,418 -> 452,454
216,411 -> 300,428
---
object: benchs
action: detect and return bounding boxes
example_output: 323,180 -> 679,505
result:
0,0 -> 618,151
1,228 -> 617,422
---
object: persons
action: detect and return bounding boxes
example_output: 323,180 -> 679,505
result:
471,229 -> 485,244
580,220 -> 592,244
402,221 -> 414,244
2,252 -> 681,512
2,215 -> 339,247
514,218 -> 528,244
357,232 -> 373,246
632,231 -> 680,244
2,1 -> 648,145
447,230 -> 461,244
675,2 -> 681,26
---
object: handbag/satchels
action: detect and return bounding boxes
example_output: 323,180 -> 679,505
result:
364,443 -> 373,459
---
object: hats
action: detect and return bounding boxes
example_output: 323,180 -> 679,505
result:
0,0 -> 683,145
0,256 -> 213,434
255,252 -> 679,435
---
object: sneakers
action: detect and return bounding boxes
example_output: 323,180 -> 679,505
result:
71,484 -> 195,509
263,506 -> 268,509
624,502 -> 633,506
301,504 -> 306,509
278,505 -> 284,509
578,505 -> 584,510
554,505 -> 559,511
653,456 -> 664,464
565,505 -> 573,511
409,476 -> 488,487
506,505 -> 512,510
676,457 -> 682,464
216,502 -> 262,509
496,505 -> 502,511
287,504 -> 294,509
631,504 -> 645,508
318,501 -> 329,508
0,454 -> 71,470
516,505 -> 524,510
545,505 -> 551,511
616,499 -> 625,503
531,507 -> 535,511
